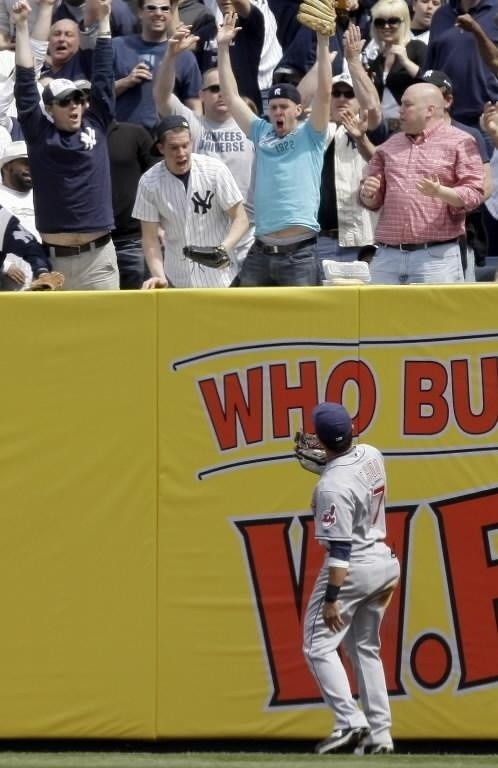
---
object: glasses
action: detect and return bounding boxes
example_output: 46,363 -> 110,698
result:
46,95 -> 81,109
332,89 -> 355,99
143,3 -> 170,13
201,84 -> 220,95
375,16 -> 401,27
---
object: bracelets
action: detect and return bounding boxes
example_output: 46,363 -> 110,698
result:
324,585 -> 341,603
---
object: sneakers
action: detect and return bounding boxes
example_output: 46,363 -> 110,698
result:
355,742 -> 394,755
315,726 -> 371,755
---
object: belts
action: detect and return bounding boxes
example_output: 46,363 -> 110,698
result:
377,237 -> 460,254
42,232 -> 112,258
255,235 -> 318,257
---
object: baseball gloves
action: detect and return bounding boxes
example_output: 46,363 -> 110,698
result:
295,432 -> 327,475
24,271 -> 64,290
297,0 -> 337,37
182,246 -> 234,269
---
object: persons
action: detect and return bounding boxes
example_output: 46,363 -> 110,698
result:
293,402 -> 402,755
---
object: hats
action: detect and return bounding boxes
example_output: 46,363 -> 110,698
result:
150,115 -> 190,157
0,140 -> 30,170
413,69 -> 454,94
43,79 -> 85,101
312,401 -> 352,443
333,73 -> 354,88
267,84 -> 302,104
